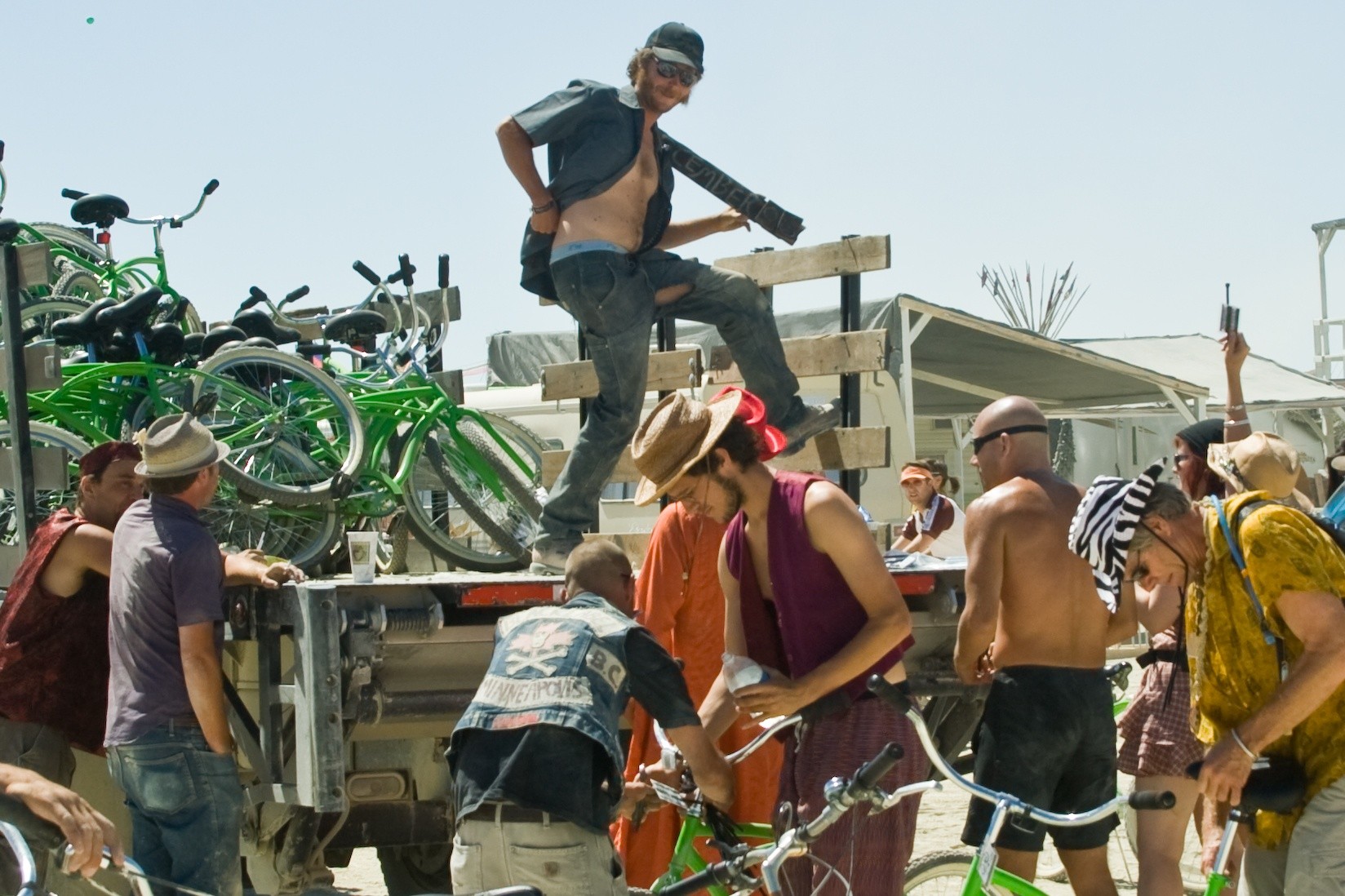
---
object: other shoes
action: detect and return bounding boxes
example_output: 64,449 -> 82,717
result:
530,538 -> 582,577
777,396 -> 842,457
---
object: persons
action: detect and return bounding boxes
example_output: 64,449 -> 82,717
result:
0,413 -> 315,896
445,391 -> 1345,896
495,20 -> 843,577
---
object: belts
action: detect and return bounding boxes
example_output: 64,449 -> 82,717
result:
464,801 -> 570,823
163,715 -> 201,728
548,239 -> 629,266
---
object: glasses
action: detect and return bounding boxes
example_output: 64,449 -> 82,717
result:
651,54 -> 695,87
668,474 -> 703,507
1122,547 -> 1149,584
971,439 -> 983,454
1172,453 -> 1191,473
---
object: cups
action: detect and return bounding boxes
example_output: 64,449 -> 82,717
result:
346,531 -> 379,582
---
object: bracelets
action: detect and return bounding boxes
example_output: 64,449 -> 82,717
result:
1224,418 -> 1250,427
529,196 -> 556,214
1231,727 -> 1258,761
1223,401 -> 1246,413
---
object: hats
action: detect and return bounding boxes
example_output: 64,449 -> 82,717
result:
133,411 -> 231,478
631,389 -> 743,507
1207,431 -> 1313,514
900,461 -> 934,486
644,21 -> 704,73
709,386 -> 787,462
1176,418 -> 1225,460
1065,457 -> 1167,615
79,441 -> 143,474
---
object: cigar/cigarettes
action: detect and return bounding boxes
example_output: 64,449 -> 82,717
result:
283,559 -> 292,574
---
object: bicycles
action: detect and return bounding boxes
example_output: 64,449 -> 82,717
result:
0,173 -> 551,581
1,669 -> 1323,896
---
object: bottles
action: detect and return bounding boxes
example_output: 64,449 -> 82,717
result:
720,653 -> 785,730
